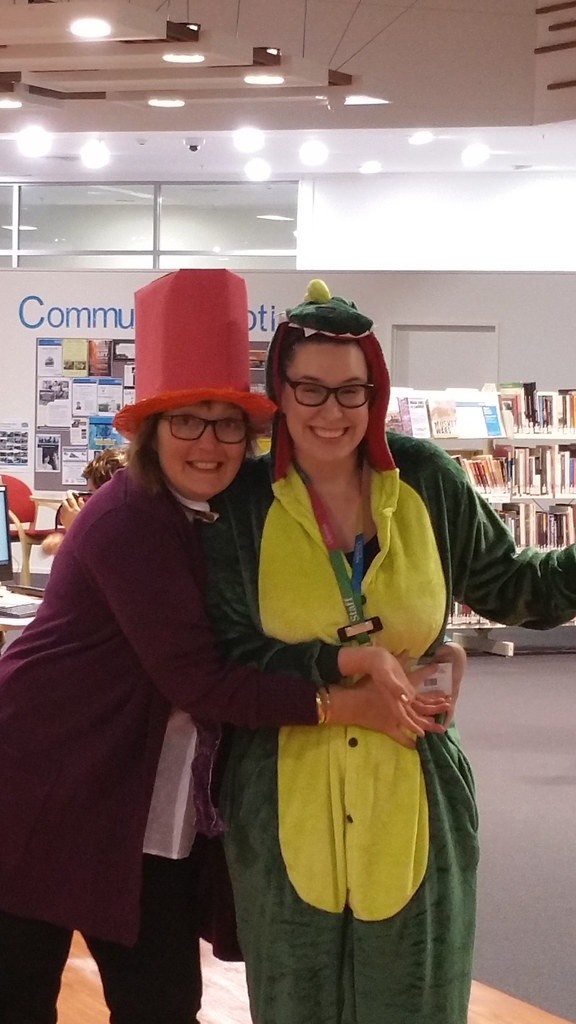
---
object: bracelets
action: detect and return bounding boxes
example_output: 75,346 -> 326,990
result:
310,679 -> 335,726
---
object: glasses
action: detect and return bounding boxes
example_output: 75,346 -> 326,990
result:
153,414 -> 250,444
279,368 -> 375,409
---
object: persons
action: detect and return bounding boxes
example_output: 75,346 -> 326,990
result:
0,264 -> 466,1023
38,445 -> 140,569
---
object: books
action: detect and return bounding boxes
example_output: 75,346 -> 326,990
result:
387,373 -> 575,625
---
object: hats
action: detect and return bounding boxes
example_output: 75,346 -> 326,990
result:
115,268 -> 276,440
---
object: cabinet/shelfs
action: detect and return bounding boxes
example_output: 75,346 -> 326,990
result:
422,431 -> 575,655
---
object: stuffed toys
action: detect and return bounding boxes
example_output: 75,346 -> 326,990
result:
199,273 -> 575,1024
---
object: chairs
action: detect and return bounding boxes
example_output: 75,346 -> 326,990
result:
1,475 -> 65,588
